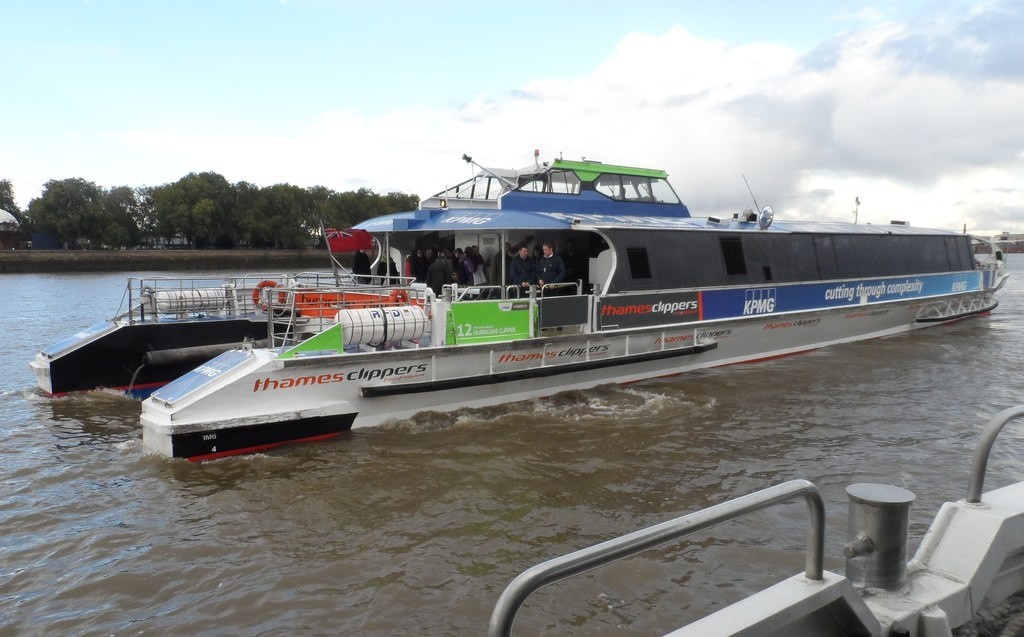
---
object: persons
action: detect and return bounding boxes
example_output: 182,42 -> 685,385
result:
615,187 -> 626,198
377,250 -> 399,286
405,236 -> 610,302
352,247 -> 373,286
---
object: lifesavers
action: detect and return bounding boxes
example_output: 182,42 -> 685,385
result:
253,281 -> 286,312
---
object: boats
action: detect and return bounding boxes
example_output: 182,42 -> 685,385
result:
29,157 -> 1014,463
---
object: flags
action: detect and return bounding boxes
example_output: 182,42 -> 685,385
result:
323,227 -> 374,253
